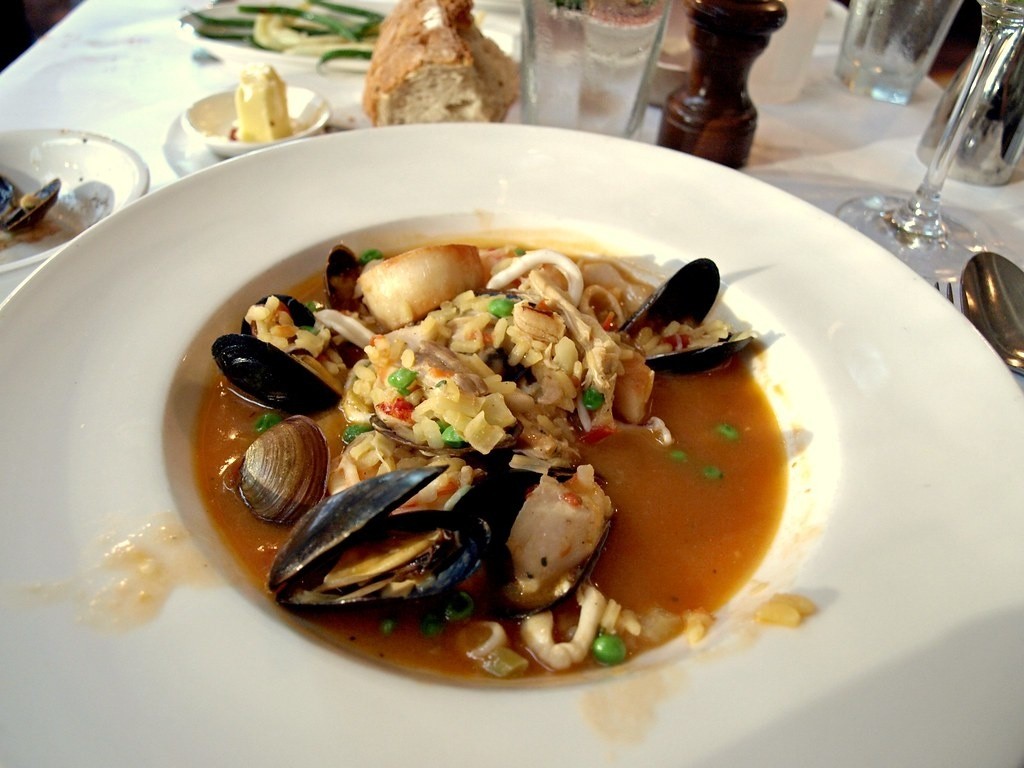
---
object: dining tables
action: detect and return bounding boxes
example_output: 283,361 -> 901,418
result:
0,0 -> 1024,768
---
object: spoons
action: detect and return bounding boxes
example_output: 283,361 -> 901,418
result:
961,252 -> 1024,377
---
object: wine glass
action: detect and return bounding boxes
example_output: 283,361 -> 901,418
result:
834,0 -> 1023,281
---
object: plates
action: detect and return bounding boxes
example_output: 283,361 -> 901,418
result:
161,110 -> 326,179
192,0 -> 520,70
0,121 -> 1023,768
181,88 -> 330,157
0,129 -> 149,271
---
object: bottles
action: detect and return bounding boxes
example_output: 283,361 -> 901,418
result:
655,0 -> 788,169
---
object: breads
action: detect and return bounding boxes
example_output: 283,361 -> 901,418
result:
363,0 -> 527,124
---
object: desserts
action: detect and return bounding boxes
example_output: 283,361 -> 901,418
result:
235,63 -> 292,142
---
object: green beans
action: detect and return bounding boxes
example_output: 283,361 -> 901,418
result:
251,250 -> 738,664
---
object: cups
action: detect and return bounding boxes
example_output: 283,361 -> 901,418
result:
835,0 -> 965,104
519,0 -> 672,140
918,0 -> 1024,187
